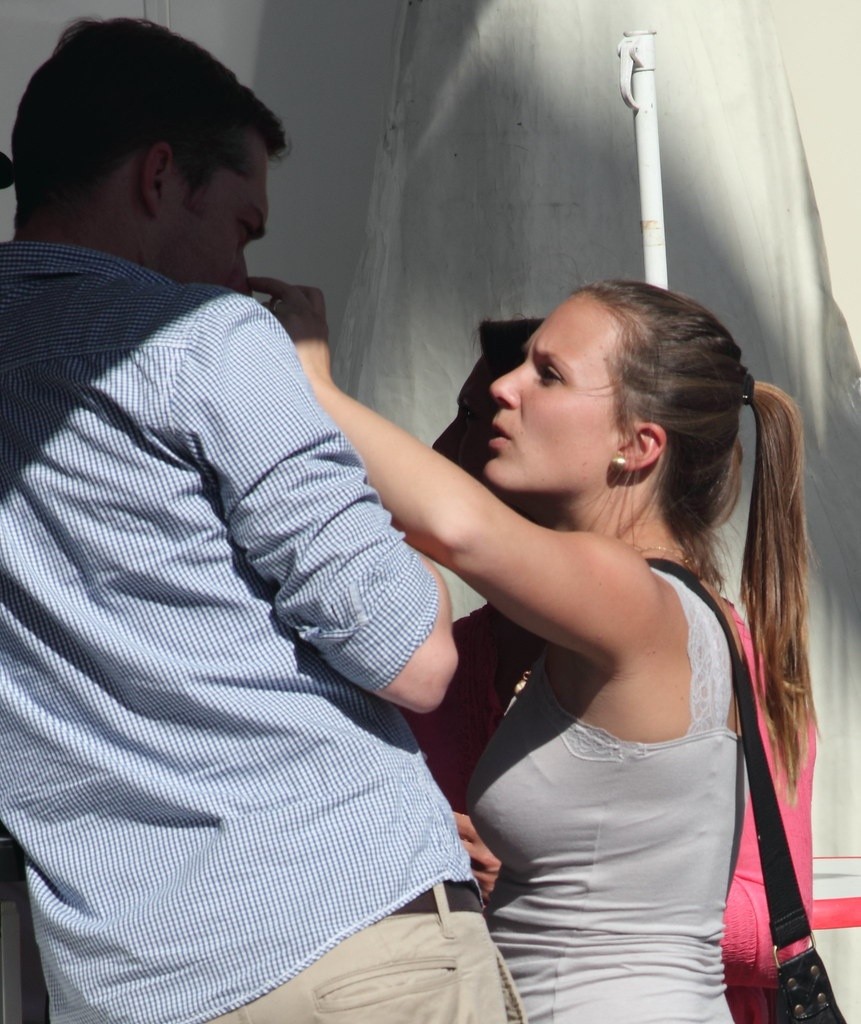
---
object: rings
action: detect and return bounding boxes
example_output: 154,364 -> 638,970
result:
273,298 -> 282,311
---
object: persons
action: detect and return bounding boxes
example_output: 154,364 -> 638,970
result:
1,15 -> 527,1024
249,275 -> 811,1024
397,317 -> 816,1024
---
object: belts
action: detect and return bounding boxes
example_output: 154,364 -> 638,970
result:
393,881 -> 482,914
